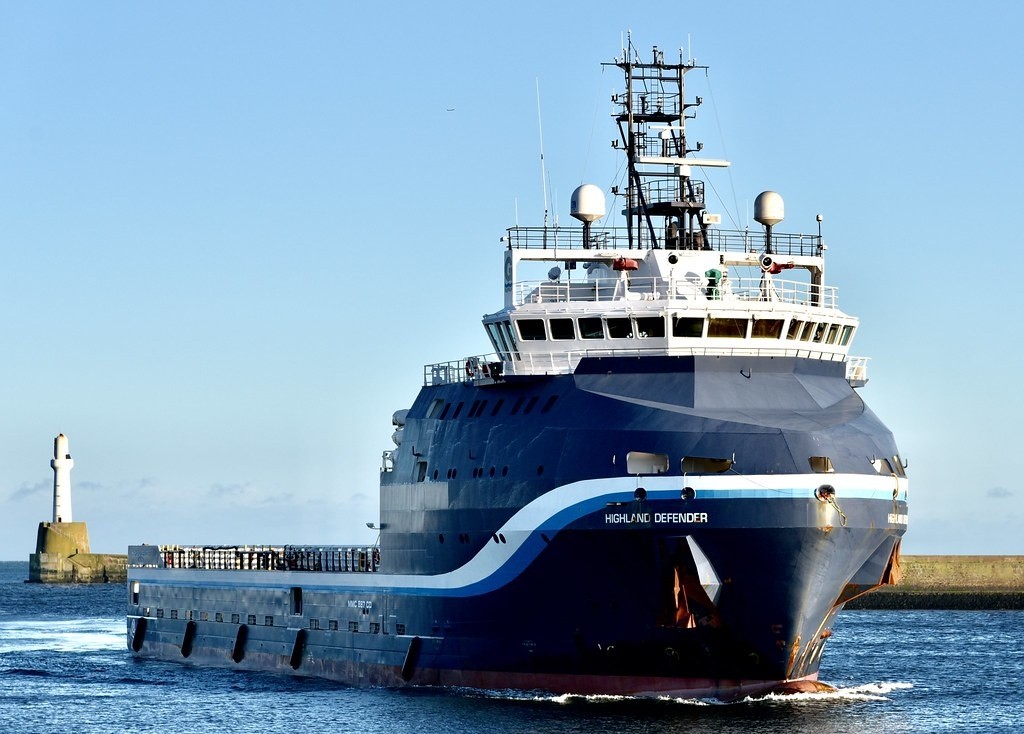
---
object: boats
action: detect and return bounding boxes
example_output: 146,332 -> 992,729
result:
127,25 -> 910,695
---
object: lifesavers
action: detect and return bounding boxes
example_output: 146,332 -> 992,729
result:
466,360 -> 491,380
